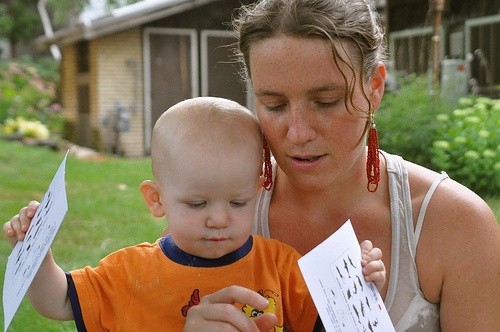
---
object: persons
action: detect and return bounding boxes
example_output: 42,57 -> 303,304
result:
161,1 -> 500,332
1,96 -> 386,332
